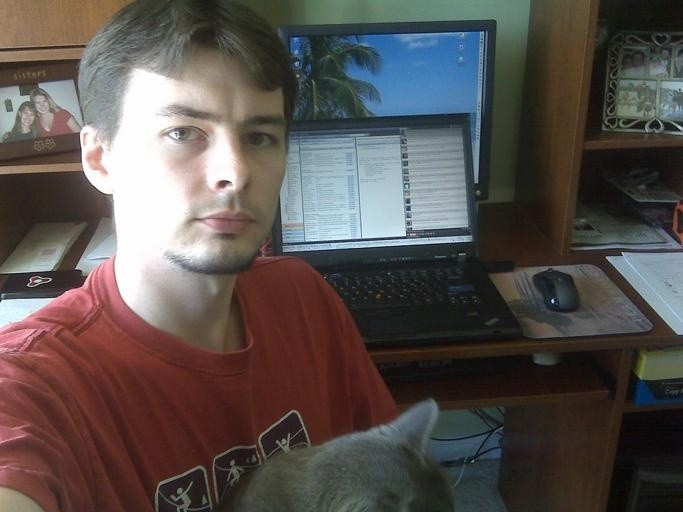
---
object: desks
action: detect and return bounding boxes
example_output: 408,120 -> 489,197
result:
333,200 -> 682,510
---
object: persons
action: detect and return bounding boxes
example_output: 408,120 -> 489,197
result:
0,0 -> 456,512
620,46 -> 683,114
3,88 -> 82,143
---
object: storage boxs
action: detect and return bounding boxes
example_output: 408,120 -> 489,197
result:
632,347 -> 682,406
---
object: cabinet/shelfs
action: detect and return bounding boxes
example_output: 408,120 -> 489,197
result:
1,49 -> 116,298
515,1 -> 682,251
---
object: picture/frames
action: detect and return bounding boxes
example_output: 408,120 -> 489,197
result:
601,29 -> 683,135
1,62 -> 81,162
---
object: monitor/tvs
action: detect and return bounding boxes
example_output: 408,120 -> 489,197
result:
276,17 -> 498,201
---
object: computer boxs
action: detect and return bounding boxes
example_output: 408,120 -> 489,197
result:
623,447 -> 683,512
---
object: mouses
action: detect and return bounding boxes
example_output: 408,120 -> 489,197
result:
532,268 -> 582,314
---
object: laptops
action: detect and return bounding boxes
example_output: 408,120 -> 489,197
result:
272,113 -> 524,350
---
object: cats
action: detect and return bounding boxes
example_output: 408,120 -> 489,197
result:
212,397 -> 455,512
